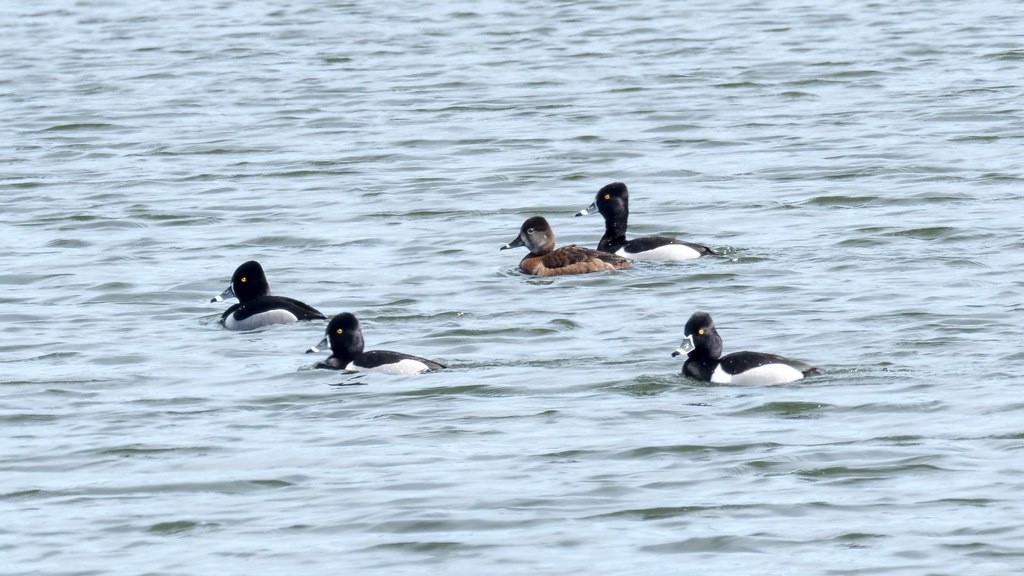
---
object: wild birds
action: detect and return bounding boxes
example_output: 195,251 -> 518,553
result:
209,259 -> 328,331
305,313 -> 445,378
499,182 -> 738,278
672,311 -> 824,389
